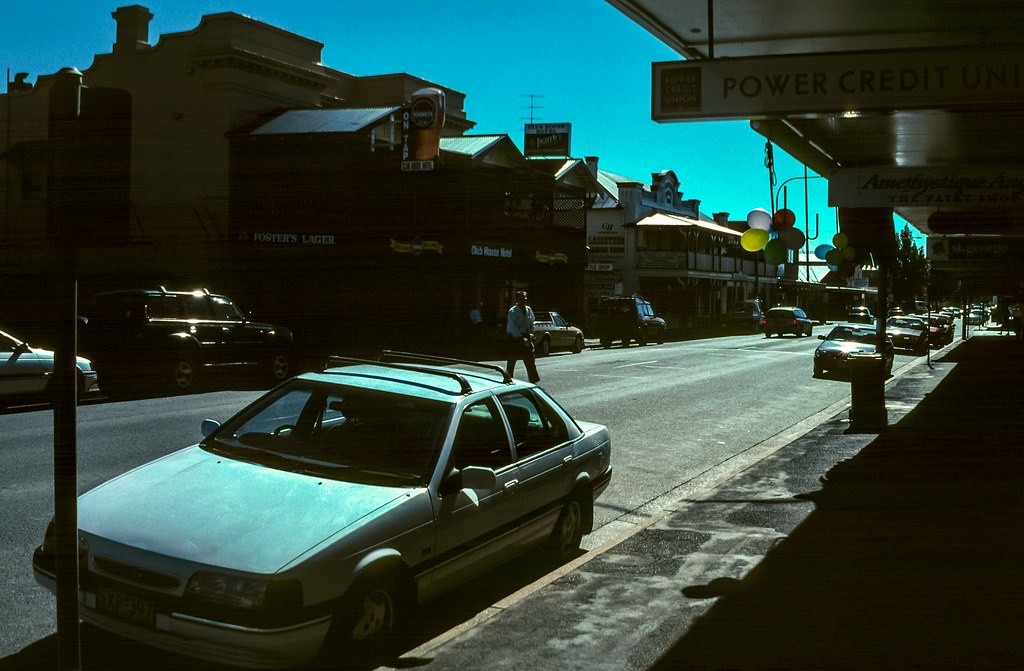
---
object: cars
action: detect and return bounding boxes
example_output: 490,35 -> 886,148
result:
30,351 -> 616,671
889,300 -> 992,346
812,322 -> 894,380
885,315 -> 931,356
0,330 -> 99,409
762,305 -> 813,339
847,305 -> 875,324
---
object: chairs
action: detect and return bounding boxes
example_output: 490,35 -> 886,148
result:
319,424 -> 367,457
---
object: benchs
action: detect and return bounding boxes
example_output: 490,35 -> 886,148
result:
366,402 -> 555,463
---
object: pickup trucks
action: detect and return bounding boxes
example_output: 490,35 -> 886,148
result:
472,309 -> 585,358
724,298 -> 766,334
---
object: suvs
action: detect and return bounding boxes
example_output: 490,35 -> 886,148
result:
596,292 -> 668,349
77,284 -> 331,397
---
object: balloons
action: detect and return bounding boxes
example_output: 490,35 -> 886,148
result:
815,233 -> 856,278
742,209 -> 805,266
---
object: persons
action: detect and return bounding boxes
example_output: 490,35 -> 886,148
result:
504,290 -> 541,384
